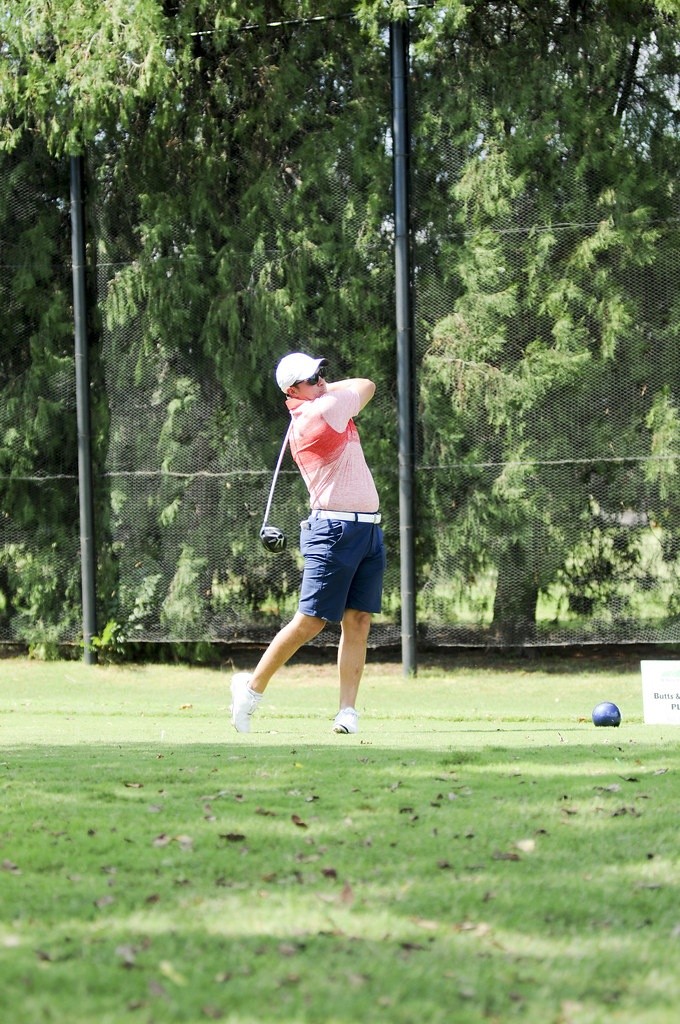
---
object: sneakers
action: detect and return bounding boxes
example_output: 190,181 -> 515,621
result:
230,672 -> 264,733
332,707 -> 360,734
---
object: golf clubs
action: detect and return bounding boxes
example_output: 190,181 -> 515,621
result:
258,419 -> 294,554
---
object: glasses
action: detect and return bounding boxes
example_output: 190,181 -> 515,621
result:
290,368 -> 326,387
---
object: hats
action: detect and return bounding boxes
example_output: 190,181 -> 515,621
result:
276,353 -> 326,394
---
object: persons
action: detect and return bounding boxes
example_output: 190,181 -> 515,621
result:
232,352 -> 386,734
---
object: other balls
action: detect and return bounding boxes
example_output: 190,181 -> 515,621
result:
592,701 -> 621,727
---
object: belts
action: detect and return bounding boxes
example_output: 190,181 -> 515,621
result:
312,509 -> 382,524
